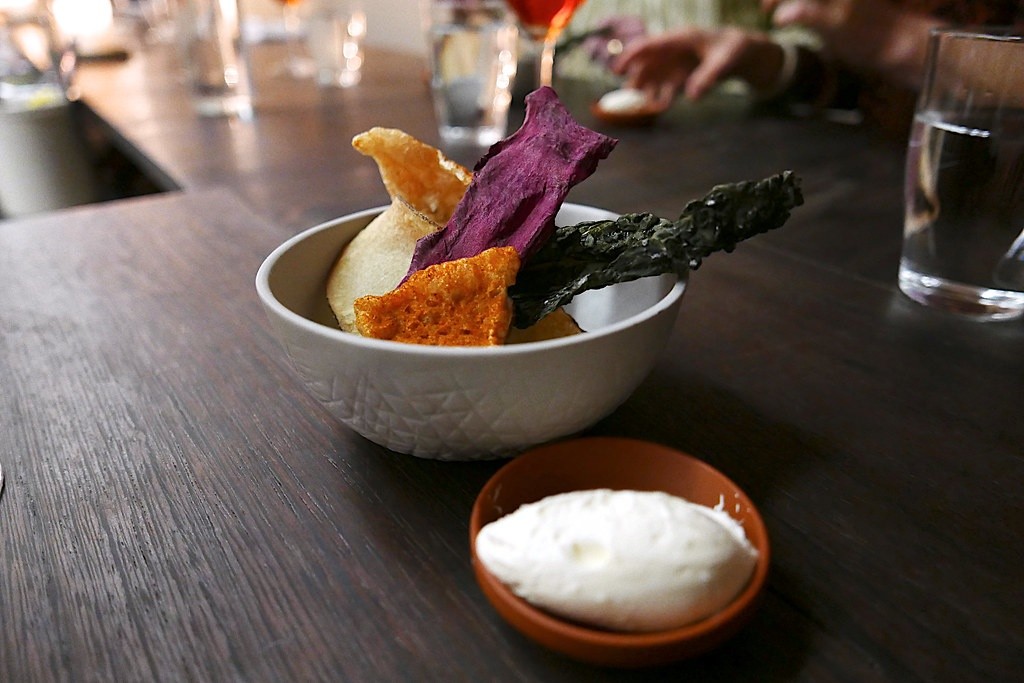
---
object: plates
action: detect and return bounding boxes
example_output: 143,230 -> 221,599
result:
468,439 -> 768,669
591,103 -> 663,120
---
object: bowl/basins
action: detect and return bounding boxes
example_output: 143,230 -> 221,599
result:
254,202 -> 690,461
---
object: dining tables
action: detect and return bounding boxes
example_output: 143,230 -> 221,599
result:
0,25 -> 1023,683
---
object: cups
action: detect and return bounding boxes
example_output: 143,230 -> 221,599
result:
426,5 -> 516,149
899,28 -> 1023,322
0,0 -> 79,110
110,0 -> 367,119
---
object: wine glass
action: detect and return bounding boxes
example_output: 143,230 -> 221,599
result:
504,0 -> 588,88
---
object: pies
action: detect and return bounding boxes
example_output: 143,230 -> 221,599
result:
353,246 -> 521,349
350,126 -> 475,228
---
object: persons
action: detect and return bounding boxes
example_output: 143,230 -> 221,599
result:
615,0 -> 1024,108
